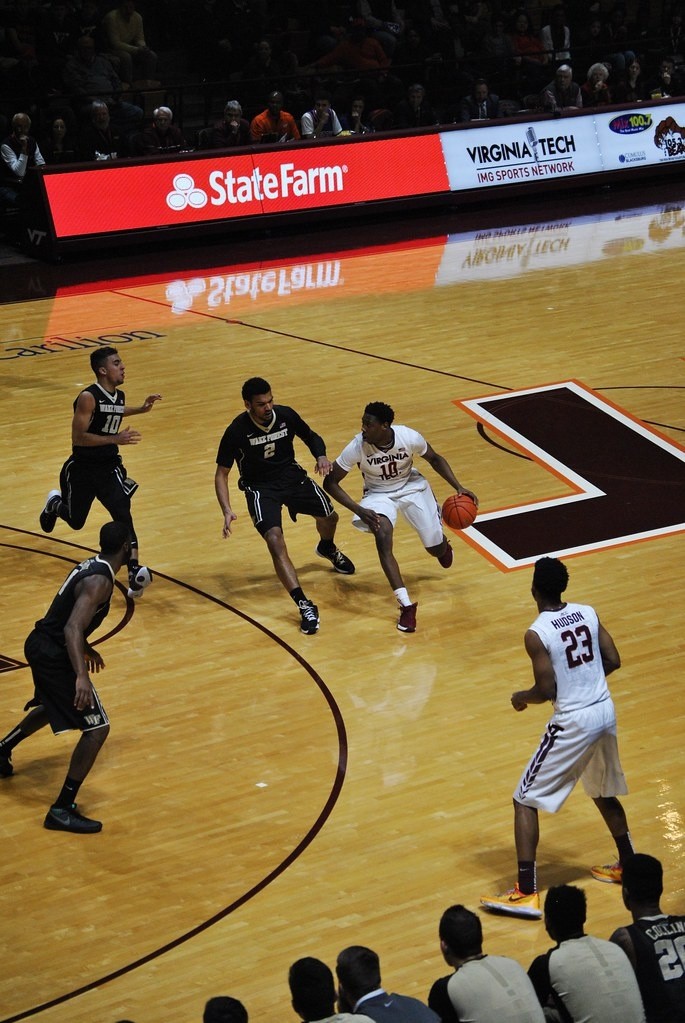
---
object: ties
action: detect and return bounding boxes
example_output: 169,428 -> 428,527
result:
479,103 -> 486,119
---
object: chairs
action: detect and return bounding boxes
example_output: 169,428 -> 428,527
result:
0,0 -> 685,240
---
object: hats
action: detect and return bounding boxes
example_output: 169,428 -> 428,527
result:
77,36 -> 96,46
352,17 -> 367,26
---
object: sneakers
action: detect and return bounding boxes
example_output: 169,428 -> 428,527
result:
39,490 -> 62,532
315,542 -> 355,574
481,882 -> 542,915
397,599 -> 417,632
128,565 -> 152,598
44,802 -> 102,832
439,539 -> 453,568
298,599 -> 320,633
0,745 -> 13,776
590,854 -> 622,884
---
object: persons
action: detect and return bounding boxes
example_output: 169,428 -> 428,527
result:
0,0 -> 685,212
215,377 -> 355,634
478,556 -> 634,921
39,346 -> 163,599
323,401 -> 478,633
0,521 -> 139,834
204,853 -> 685,1023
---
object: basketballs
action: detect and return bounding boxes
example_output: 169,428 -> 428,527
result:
442,494 -> 478,530
